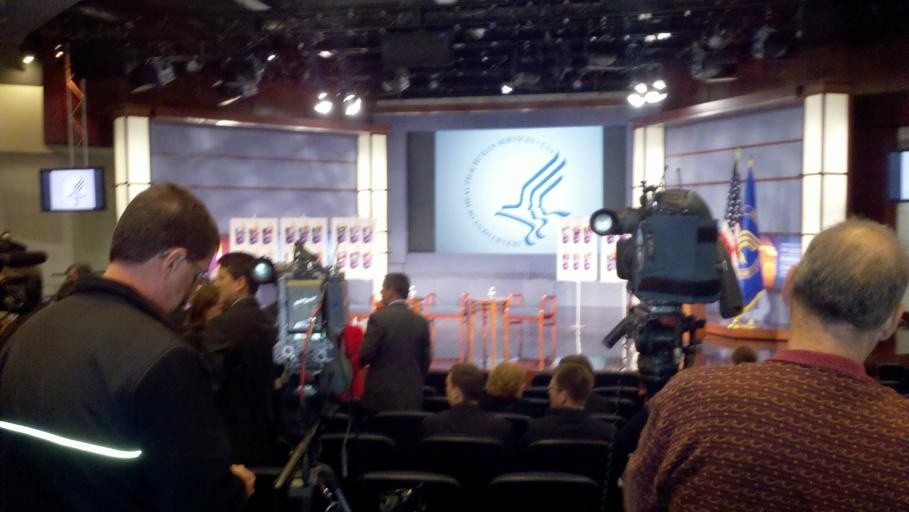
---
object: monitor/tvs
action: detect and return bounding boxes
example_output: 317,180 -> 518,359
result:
40,167 -> 105,212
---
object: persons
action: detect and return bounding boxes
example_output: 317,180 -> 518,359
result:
417,355 -> 617,443
620,213 -> 908,512
182,284 -> 224,349
334,323 -> 365,400
358,271 -> 433,412
204,253 -> 278,467
730,345 -> 758,366
61,262 -> 91,281
0,179 -> 256,512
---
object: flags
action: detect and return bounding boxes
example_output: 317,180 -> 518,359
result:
734,169 -> 767,320
719,169 -> 742,272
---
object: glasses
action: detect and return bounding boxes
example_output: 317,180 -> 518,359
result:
159,251 -> 209,288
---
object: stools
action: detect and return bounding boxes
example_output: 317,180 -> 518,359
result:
350,293 -> 557,373
